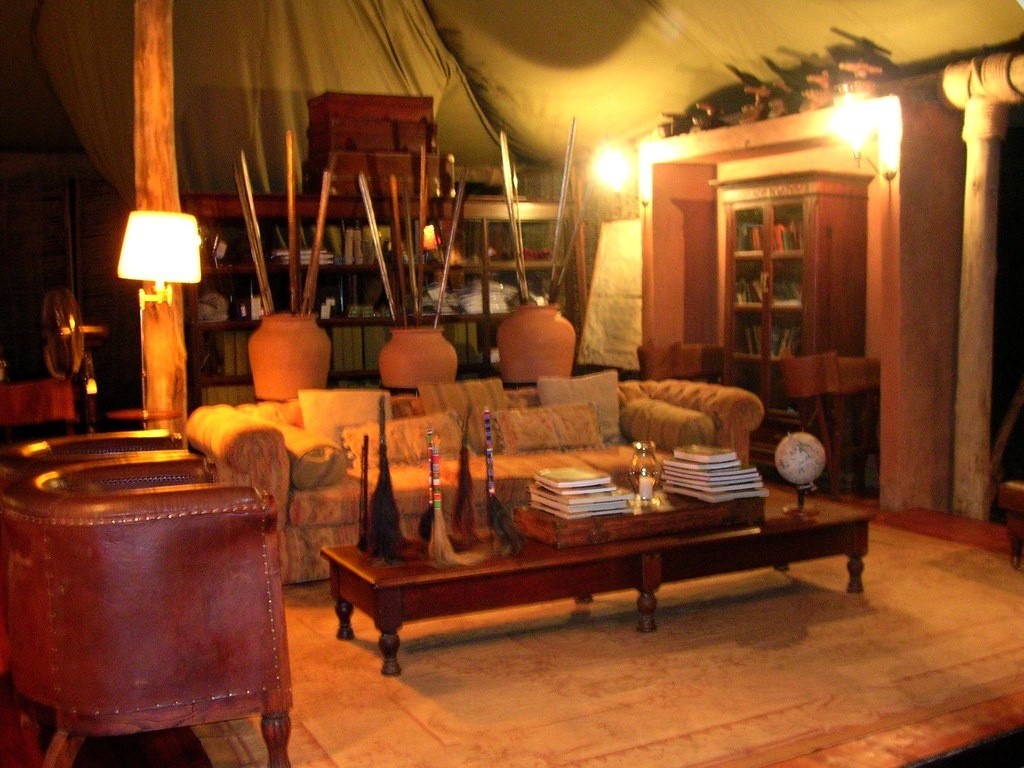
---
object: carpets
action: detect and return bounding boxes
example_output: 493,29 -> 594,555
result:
193,505 -> 1024,768
871,507 -> 1010,554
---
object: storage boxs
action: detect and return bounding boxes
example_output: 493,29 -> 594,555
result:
307,121 -> 440,151
308,90 -> 438,121
302,148 -> 447,198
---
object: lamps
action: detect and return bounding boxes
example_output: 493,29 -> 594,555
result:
115,208 -> 203,309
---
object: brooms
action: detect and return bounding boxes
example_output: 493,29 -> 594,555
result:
483,407 -> 527,555
423,401 -> 487,569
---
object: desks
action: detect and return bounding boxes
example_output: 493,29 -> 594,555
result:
0,378 -> 78,442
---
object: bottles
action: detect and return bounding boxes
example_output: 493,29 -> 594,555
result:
628,441 -> 661,500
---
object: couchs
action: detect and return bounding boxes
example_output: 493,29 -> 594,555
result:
186,368 -> 772,588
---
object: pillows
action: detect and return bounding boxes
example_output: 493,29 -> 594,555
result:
490,398 -> 607,457
528,371 -> 623,449
340,407 -> 460,470
297,384 -> 387,442
276,421 -> 350,490
620,396 -> 717,454
417,378 -> 507,450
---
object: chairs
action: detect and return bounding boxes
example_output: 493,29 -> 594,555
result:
0,428 -> 189,464
0,453 -> 298,768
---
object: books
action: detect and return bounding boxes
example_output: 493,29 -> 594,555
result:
528,461 -> 636,520
736,261 -> 801,306
663,444 -> 769,504
745,323 -> 802,359
737,217 -> 802,251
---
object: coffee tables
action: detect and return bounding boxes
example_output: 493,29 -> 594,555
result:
319,483 -> 879,676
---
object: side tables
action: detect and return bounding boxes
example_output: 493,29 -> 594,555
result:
108,408 -> 182,430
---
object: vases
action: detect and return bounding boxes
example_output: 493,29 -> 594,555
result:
249,309 -> 332,400
378,325 -> 460,390
497,303 -> 577,382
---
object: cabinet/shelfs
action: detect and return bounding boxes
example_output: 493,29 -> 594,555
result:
718,167 -> 874,497
184,193 -> 588,409
